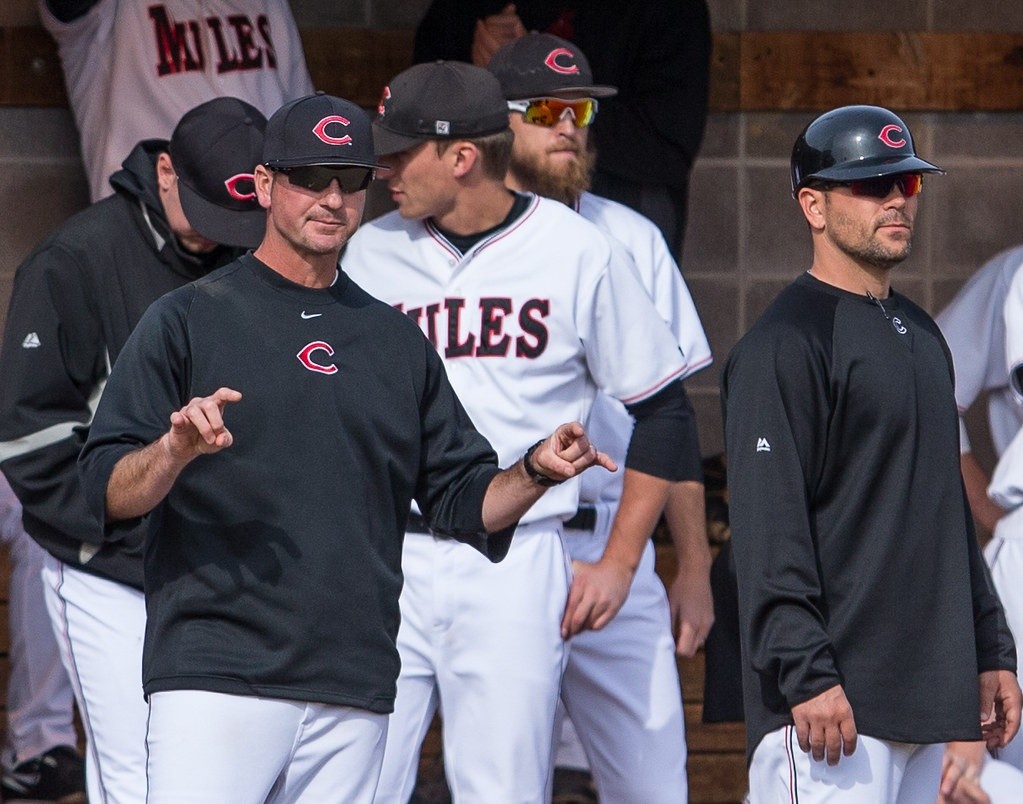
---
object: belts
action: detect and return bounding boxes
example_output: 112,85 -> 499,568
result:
562,508 -> 595,530
404,512 -> 434,536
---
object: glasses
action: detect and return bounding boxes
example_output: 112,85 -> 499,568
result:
811,169 -> 923,198
506,96 -> 598,129
268,165 -> 376,194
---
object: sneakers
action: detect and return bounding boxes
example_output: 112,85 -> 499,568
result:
0,746 -> 87,804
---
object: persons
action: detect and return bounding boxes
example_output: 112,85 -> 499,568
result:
0,0 -> 715,804
720,106 -> 1023,804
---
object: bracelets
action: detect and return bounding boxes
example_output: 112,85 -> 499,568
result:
524,438 -> 565,488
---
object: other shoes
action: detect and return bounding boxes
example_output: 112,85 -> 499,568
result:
413,766 -> 451,804
550,767 -> 596,804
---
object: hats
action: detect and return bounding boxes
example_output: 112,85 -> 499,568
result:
169,97 -> 269,248
371,61 -> 511,157
261,92 -> 391,171
487,31 -> 618,100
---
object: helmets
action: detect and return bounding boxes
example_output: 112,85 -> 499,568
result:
791,105 -> 946,201
